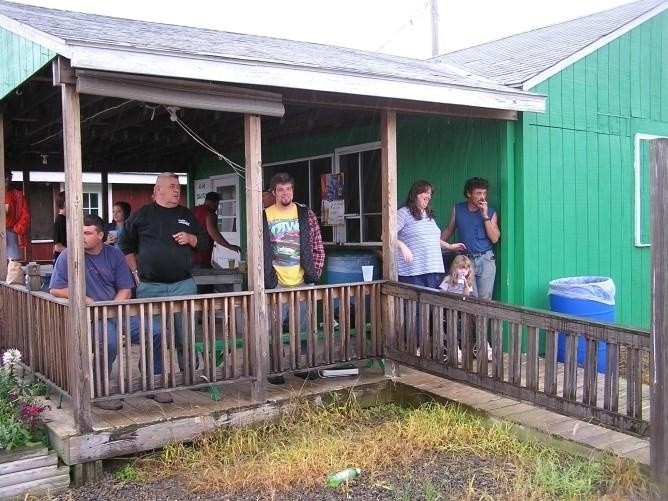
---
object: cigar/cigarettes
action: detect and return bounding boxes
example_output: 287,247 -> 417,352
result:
480,200 -> 488,204
171,234 -> 182,238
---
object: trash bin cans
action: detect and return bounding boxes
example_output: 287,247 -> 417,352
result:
550,276 -> 615,378
327,249 -> 383,329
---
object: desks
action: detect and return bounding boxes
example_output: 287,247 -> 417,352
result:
190,268 -> 248,334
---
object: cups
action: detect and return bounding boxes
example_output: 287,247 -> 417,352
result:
457,272 -> 465,289
238,261 -> 246,272
109,231 -> 117,240
228,259 -> 235,269
362,266 -> 374,281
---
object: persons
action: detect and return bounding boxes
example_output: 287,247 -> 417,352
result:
438,176 -> 501,360
47,214 -> 173,411
188,190 -> 243,325
261,187 -> 276,208
381,178 -> 468,357
101,200 -> 132,247
3,168 -> 31,259
51,189 -> 66,266
438,254 -> 473,364
261,171 -> 326,385
116,171 -> 210,393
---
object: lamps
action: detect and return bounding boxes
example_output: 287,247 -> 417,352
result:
41,154 -> 48,165
167,106 -> 180,122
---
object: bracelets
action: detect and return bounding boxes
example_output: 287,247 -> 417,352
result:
481,215 -> 490,220
130,270 -> 138,273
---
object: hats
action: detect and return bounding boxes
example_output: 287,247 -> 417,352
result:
206,192 -> 224,200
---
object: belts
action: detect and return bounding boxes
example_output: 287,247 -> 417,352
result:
466,250 -> 486,257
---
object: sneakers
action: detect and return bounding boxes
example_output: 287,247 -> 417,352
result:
488,344 -> 492,360
146,392 -> 173,403
294,371 -> 317,380
94,398 -> 123,410
458,348 -> 462,363
268,375 -> 285,385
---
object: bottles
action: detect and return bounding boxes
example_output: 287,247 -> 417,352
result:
326,468 -> 362,487
